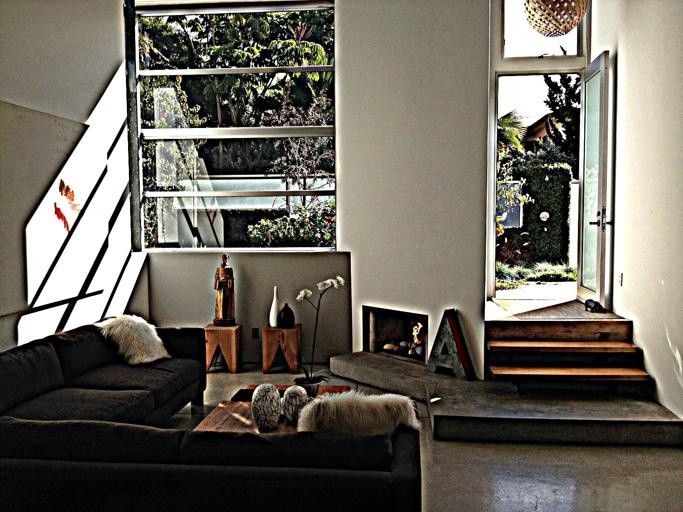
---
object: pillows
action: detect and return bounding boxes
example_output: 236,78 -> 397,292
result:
298,390 -> 421,433
94,315 -> 172,364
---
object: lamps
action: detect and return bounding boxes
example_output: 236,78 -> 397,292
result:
520,2 -> 593,43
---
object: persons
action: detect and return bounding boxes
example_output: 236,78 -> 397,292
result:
213,253 -> 234,320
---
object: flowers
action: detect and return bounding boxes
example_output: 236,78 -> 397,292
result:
298,273 -> 344,386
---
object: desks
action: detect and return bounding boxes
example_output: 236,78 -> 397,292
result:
194,400 -> 298,434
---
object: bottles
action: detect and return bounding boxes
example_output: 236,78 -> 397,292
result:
269,286 -> 280,327
277,303 -> 295,329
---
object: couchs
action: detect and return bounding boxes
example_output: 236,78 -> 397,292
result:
2,401 -> 422,512
1,316 -> 207,428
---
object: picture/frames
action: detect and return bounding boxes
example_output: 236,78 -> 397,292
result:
357,302 -> 429,366
